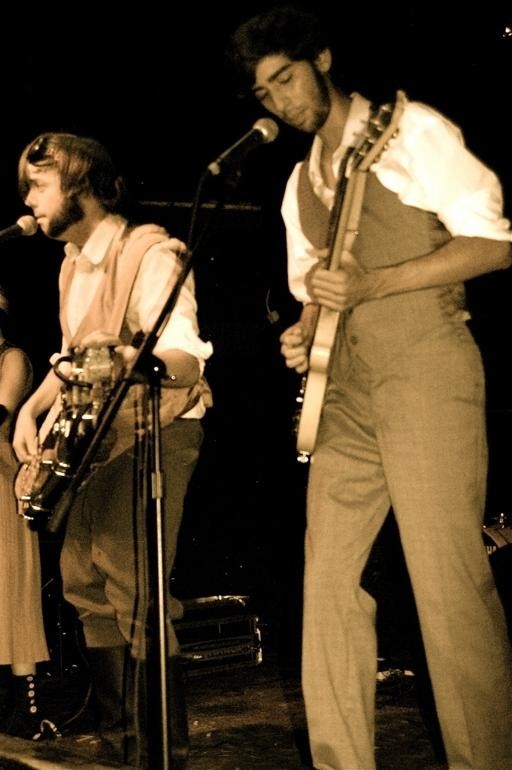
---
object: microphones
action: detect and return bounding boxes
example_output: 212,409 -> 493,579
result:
210,117 -> 281,181
0,213 -> 39,240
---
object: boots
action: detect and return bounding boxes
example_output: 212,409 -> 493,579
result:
120,658 -> 189,768
32,646 -> 126,742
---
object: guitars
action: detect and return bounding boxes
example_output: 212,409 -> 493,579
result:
13,342 -> 118,520
296,90 -> 404,455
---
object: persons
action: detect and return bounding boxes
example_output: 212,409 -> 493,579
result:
228,13 -> 512,770
12,130 -> 215,769
0,293 -> 52,743
